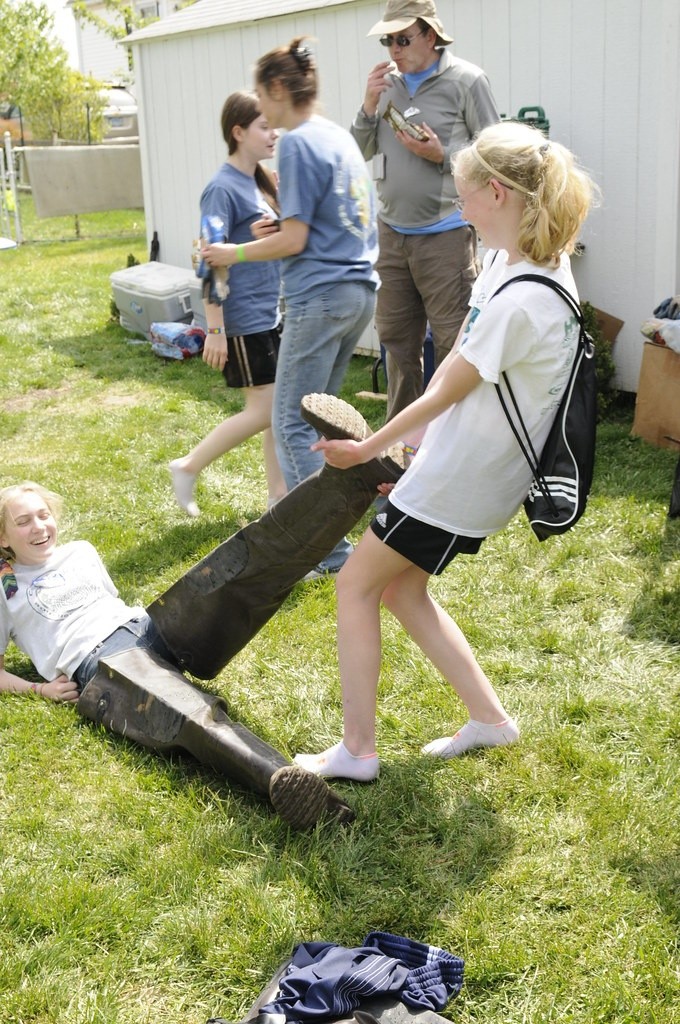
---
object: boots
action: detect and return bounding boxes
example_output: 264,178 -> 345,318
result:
269,393 -> 410,565
206,721 -> 353,832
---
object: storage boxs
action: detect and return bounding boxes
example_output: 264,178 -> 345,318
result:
110,260 -> 196,338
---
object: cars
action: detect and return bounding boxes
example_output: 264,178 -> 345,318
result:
78,88 -> 139,138
0,99 -> 38,171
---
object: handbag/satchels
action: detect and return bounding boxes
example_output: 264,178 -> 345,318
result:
630,339 -> 680,454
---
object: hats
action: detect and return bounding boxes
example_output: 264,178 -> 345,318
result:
366,1 -> 454,46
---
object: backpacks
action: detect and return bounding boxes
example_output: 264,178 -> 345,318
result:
476,273 -> 598,542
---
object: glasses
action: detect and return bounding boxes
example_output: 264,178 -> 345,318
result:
380,26 -> 431,47
452,179 -> 513,213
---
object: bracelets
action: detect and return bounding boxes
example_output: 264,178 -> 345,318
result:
236,243 -> 245,264
206,327 -> 226,334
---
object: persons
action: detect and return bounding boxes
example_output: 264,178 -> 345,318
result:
349,1 -> 501,428
167,35 -> 382,586
295,123 -> 601,783
1,393 -> 421,833
